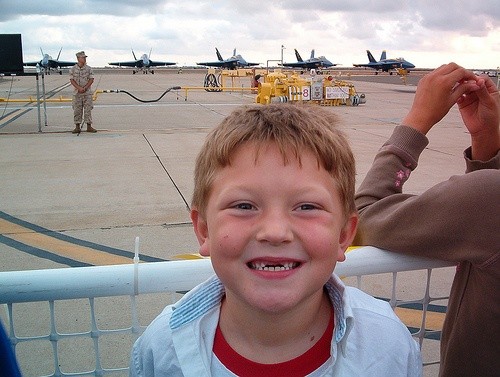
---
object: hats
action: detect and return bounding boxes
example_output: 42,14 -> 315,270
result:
76,51 -> 89,58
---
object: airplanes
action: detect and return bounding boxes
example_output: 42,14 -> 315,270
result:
353,50 -> 415,76
23,45 -> 78,75
196,47 -> 260,70
278,48 -> 337,74
108,47 -> 178,74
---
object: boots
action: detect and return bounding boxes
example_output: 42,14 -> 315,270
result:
72,123 -> 82,133
86,124 -> 98,133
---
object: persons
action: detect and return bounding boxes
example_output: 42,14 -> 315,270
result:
129,104 -> 423,377
355,62 -> 500,377
69,51 -> 97,133
